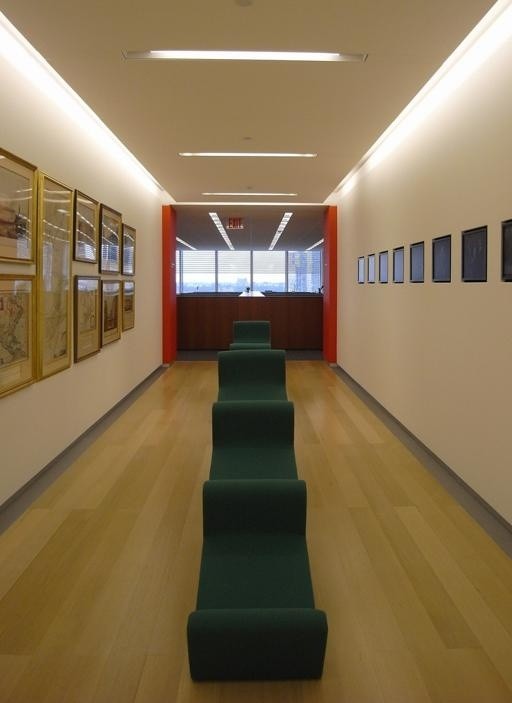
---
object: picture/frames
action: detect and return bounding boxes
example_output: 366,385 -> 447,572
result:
357,217 -> 512,284
0,146 -> 137,400
